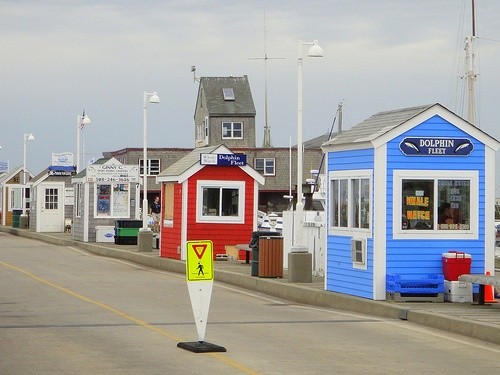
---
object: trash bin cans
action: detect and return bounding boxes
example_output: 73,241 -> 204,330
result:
259,236 -> 284,277
114,220 -> 143,245
249,232 -> 282,276
12,210 -> 23,227
65,218 -> 71,226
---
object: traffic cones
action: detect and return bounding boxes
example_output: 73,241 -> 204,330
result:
483,272 -> 498,303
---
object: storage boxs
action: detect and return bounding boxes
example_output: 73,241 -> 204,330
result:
441,251 -> 471,281
443,280 -> 473,303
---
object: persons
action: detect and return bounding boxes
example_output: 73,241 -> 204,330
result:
438,203 -> 453,224
150,196 -> 161,233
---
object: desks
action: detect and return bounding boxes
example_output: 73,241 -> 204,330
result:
236,244 -> 252,264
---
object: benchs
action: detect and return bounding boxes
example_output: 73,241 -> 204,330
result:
458,272 -> 500,304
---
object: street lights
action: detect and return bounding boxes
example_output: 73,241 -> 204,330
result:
137,90 -> 160,251
287,39 -> 324,282
76,115 -> 91,175
18,133 -> 34,229
